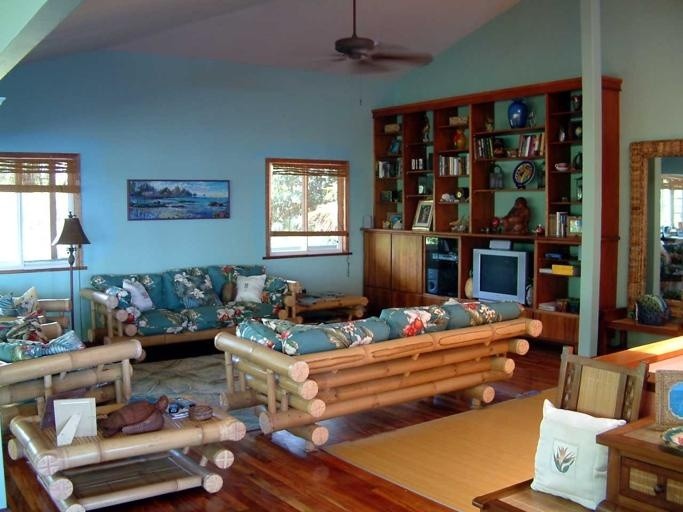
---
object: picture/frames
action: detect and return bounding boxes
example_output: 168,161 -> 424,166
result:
126,178 -> 232,220
41,388 -> 86,430
54,398 -> 97,447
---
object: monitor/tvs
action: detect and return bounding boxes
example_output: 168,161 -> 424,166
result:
471,248 -> 533,305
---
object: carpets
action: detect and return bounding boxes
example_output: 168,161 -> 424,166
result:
321,386 -> 556,511
130,353 -> 262,432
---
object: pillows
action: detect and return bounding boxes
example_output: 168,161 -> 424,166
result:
261,318 -> 294,333
122,279 -> 154,312
13,287 -> 48,319
530,399 -> 626,510
4,321 -> 53,346
236,275 -> 266,303
176,275 -> 222,308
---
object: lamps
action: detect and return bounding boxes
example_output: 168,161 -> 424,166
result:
53,212 -> 91,329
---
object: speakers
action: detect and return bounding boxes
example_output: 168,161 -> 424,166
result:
426,266 -> 454,296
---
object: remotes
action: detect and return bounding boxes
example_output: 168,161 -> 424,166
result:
175,396 -> 196,410
168,401 -> 179,414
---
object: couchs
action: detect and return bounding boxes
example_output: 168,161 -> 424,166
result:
0,296 -> 140,436
215,302 -> 542,446
81,265 -> 303,362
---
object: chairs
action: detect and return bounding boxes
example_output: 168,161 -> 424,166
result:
474,347 -> 649,511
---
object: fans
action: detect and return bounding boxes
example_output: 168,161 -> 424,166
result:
305,0 -> 433,82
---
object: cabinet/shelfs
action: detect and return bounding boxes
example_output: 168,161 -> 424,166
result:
594,416 -> 681,512
360,73 -> 623,356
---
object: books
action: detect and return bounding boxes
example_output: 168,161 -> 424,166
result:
165,396 -> 195,421
549,211 -> 568,237
438,154 -> 469,176
473,132 -> 545,158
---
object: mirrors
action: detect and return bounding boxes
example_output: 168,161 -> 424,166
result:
628,139 -> 683,317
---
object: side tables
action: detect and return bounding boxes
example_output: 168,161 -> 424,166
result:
298,291 -> 368,321
614,315 -> 683,348
